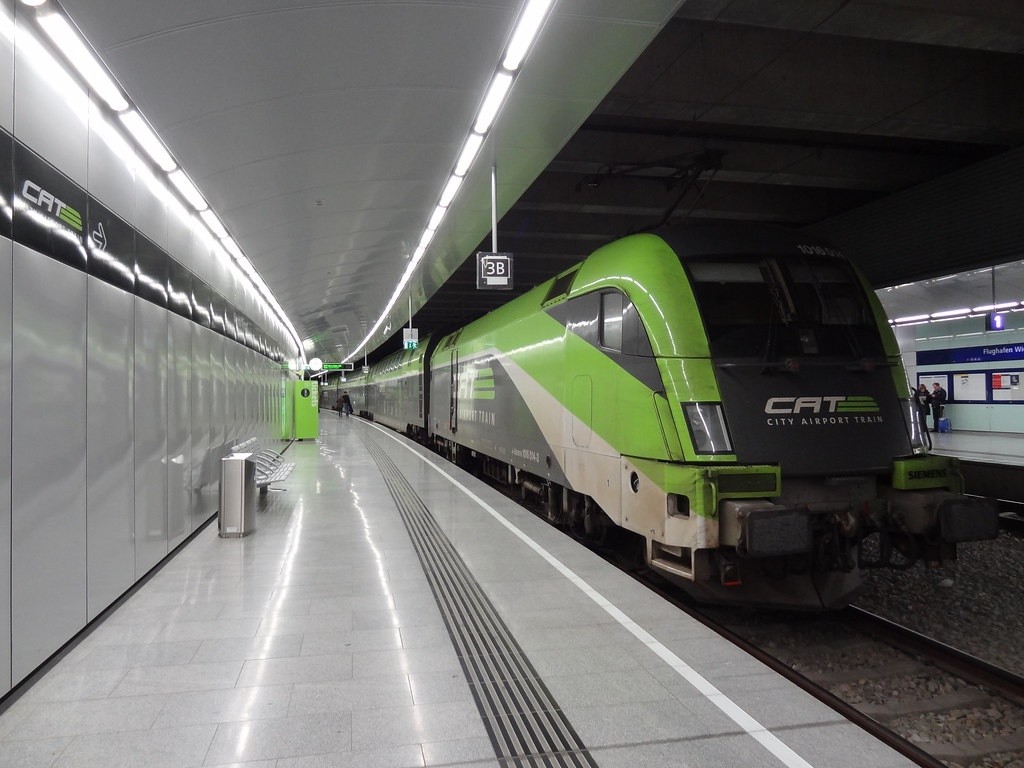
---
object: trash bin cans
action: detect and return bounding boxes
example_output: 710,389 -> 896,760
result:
218,452 -> 259,539
144,452 -> 188,540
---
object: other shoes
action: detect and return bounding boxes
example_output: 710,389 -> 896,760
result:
930,428 -> 939,433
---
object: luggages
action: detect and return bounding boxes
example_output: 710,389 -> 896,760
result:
938,404 -> 951,434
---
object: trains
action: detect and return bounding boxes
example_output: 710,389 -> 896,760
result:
319,237 -> 967,615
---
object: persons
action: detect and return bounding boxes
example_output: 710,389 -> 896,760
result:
342,391 -> 350,416
336,395 -> 345,417
928,383 -> 946,433
914,385 -> 931,424
